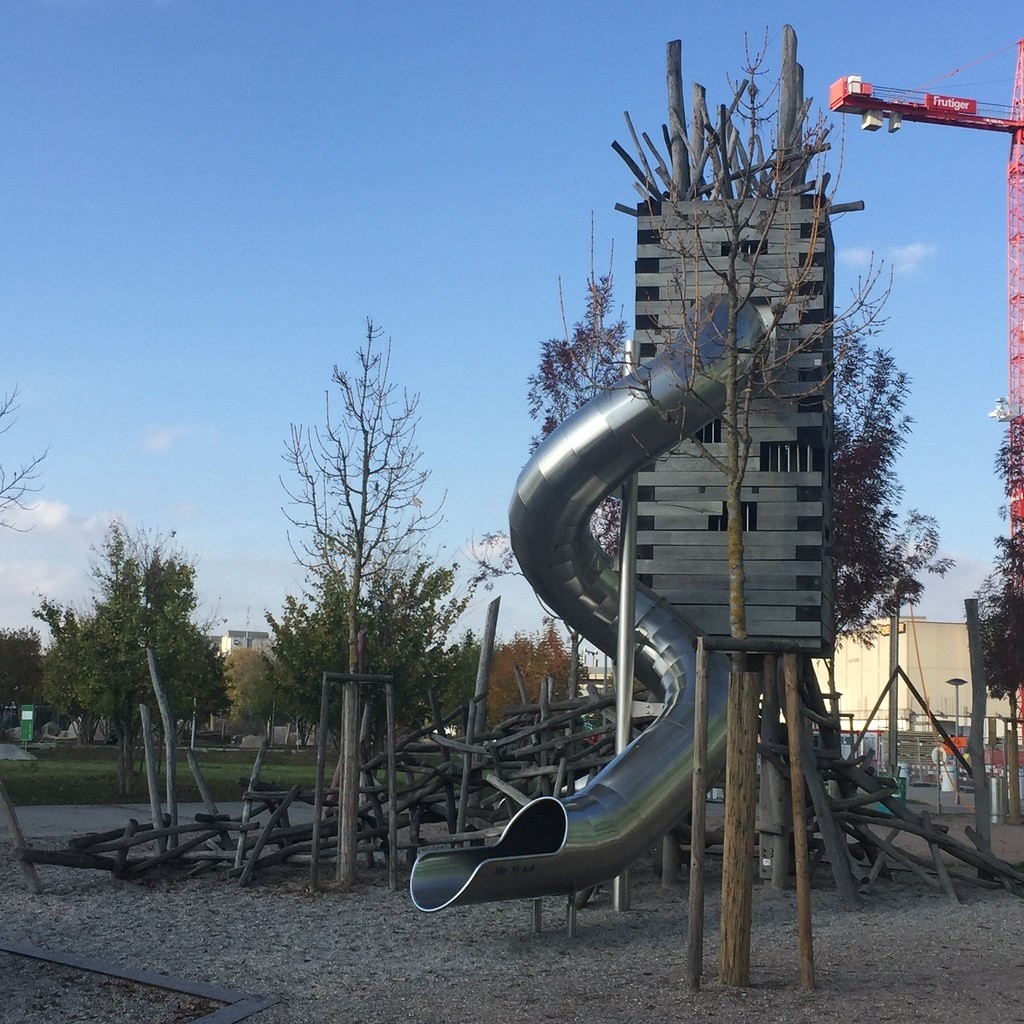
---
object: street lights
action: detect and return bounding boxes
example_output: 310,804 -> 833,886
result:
945,679 -> 969,807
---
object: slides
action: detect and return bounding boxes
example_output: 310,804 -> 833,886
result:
409,293 -> 772,913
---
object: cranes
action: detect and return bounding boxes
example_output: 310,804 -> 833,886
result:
828,33 -> 1024,767
